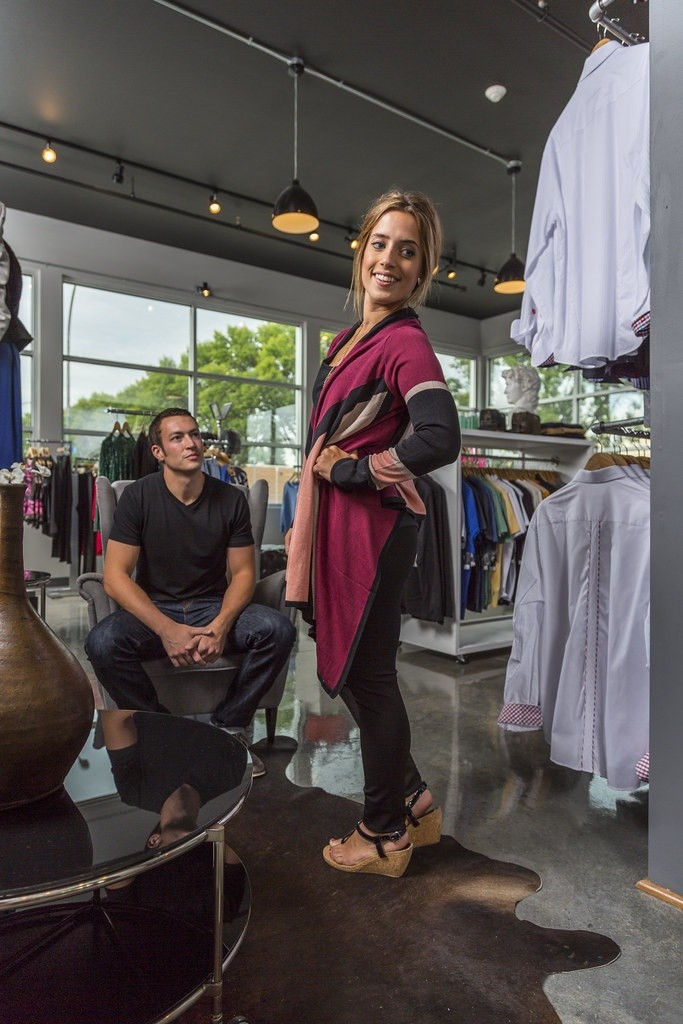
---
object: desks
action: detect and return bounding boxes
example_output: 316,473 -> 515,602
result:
24,571 -> 52,621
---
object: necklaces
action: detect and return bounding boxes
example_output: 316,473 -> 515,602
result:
323,321 -> 369,387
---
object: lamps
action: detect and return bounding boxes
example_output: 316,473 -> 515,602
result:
308,229 -> 318,243
344,230 -> 357,249
112,162 -> 125,185
42,141 -> 57,163
477,271 -> 488,285
208,192 -> 221,215
195,282 -> 210,299
445,269 -> 456,278
269,63 -> 319,235
494,166 -> 527,294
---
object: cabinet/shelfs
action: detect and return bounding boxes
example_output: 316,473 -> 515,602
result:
398,428 -> 592,663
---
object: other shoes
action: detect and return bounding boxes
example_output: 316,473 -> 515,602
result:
233,733 -> 266,777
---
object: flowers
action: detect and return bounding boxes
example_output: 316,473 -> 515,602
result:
0,462 -> 54,486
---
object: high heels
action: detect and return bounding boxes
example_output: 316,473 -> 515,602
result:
341,781 -> 441,847
323,819 -> 413,878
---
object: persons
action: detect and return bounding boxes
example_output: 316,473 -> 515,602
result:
96,710 -> 247,854
499,364 -> 541,413
104,845 -> 244,928
282,187 -> 462,882
84,407 -> 296,776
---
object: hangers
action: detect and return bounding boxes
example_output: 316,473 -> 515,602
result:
24,406 -> 235,477
288,464 -> 303,485
588,18 -> 645,57
461,454 -> 566,488
584,427 -> 652,473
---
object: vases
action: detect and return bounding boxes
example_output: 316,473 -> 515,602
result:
0,485 -> 94,805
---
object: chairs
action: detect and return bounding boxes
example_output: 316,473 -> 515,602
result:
77,477 -> 298,759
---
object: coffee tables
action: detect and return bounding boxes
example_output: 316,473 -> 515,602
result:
0,709 -> 253,1024
